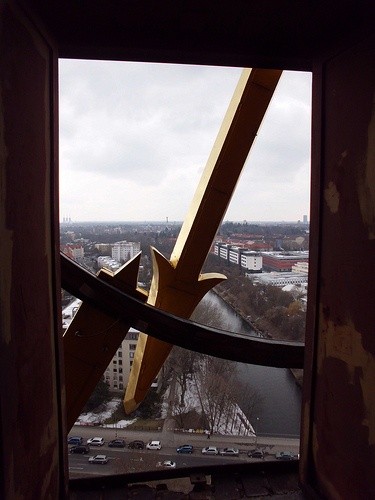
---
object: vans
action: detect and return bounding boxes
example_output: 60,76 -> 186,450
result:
68,436 -> 82,445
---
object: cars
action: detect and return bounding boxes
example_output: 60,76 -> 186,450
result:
276,451 -> 294,460
247,449 -> 265,459
128,440 -> 146,449
69,446 -> 90,454
87,437 -> 104,446
219,448 -> 239,456
108,440 -> 125,450
146,441 -> 161,450
155,461 -> 176,469
176,445 -> 193,454
202,446 -> 218,456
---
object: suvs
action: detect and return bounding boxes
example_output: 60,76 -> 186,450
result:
89,455 -> 108,465
